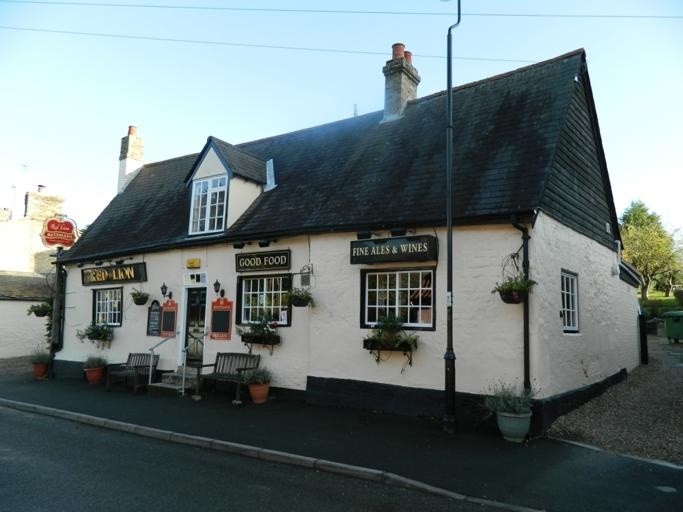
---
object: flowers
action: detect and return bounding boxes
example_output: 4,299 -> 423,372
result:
478,378 -> 544,416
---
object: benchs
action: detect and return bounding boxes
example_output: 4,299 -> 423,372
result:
191,351 -> 259,405
106,352 -> 159,392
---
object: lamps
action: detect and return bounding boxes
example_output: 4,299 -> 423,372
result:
160,282 -> 171,299
213,279 -> 223,297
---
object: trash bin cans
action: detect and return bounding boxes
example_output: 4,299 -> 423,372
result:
663,311 -> 683,344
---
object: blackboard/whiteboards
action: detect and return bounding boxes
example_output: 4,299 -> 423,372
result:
162,300 -> 177,338
147,300 -> 162,336
211,298 -> 233,340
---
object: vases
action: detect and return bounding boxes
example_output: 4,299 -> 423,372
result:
495,406 -> 533,444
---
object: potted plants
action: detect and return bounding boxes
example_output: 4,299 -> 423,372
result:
83,356 -> 105,384
245,368 -> 273,402
75,320 -> 113,349
129,286 -> 149,305
26,303 -> 49,318
30,348 -> 49,377
365,312 -> 419,374
279,288 -> 315,308
237,312 -> 278,345
491,270 -> 536,305
